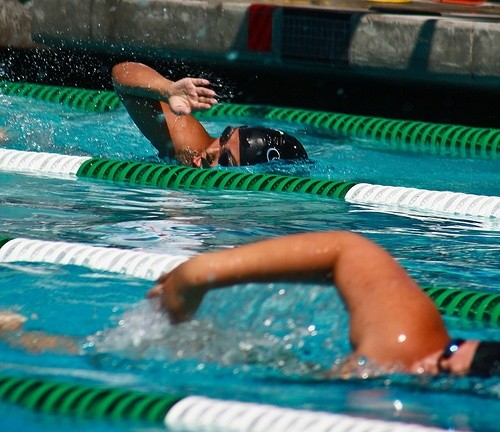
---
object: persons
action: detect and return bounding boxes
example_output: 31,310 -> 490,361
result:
0,62 -> 311,176
0,230 -> 500,400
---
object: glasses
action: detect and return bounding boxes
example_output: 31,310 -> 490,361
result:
436,338 -> 465,378
218,125 -> 237,167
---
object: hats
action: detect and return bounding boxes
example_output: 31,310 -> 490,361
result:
239,126 -> 308,166
467,340 -> 500,378
